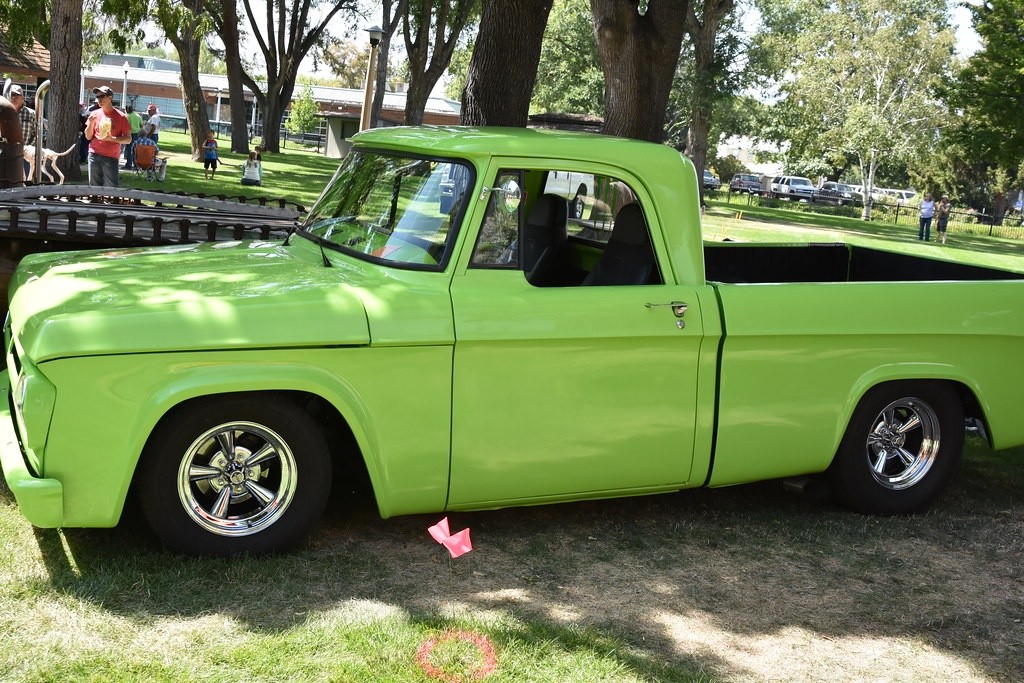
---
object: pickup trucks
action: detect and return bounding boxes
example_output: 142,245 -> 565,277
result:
0,125 -> 1024,559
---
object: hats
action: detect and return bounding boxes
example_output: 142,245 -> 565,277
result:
9,85 -> 23,95
942,195 -> 949,199
147,104 -> 156,111
93,85 -> 113,97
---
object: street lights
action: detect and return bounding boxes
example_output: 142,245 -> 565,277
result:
360,25 -> 388,131
121,60 -> 130,110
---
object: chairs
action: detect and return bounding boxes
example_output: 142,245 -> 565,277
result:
580,200 -> 656,286
133,143 -> 170,182
516,193 -> 568,286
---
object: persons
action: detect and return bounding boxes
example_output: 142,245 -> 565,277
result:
202,129 -> 219,180
935,195 -> 952,244
241,146 -> 263,185
83,86 -> 132,189
10,84 -> 48,186
79,99 -> 160,181
917,193 -> 937,242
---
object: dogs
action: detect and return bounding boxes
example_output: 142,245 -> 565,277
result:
23,143 -> 77,186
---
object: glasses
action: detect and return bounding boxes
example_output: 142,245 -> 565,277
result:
95,95 -> 106,99
11,93 -> 19,96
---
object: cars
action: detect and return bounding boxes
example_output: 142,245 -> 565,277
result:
731,173 -> 764,195
702,169 -> 718,191
762,176 -> 918,206
